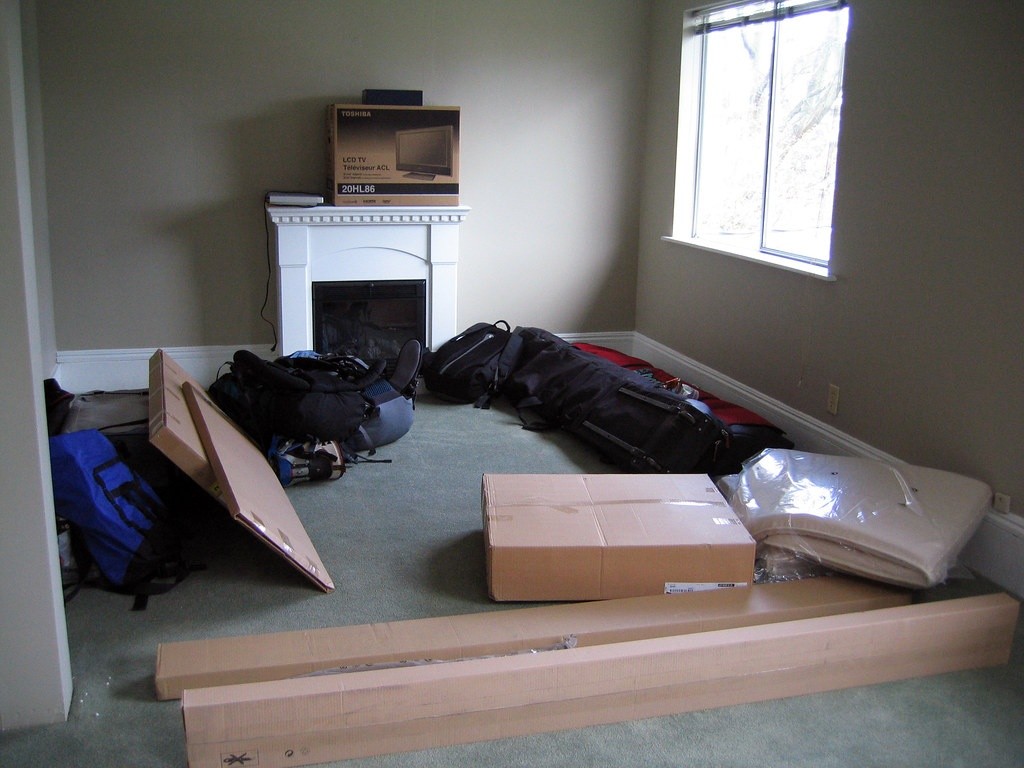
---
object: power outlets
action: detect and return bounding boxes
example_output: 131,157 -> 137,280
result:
825,384 -> 840,416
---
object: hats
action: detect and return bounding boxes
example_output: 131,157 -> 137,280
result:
44,378 -> 75,412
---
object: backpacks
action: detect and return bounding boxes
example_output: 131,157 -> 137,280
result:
421,320 -> 523,409
295,354 -> 414,448
46,428 -> 198,612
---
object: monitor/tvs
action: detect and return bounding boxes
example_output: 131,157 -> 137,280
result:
396,125 -> 453,180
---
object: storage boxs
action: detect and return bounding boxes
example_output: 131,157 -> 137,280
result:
480,473 -> 757,603
149,348 -> 232,510
322,103 -> 460,207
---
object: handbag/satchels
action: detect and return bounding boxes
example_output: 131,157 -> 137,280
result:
207,349 -> 365,442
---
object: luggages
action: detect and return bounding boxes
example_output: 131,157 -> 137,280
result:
568,371 -> 736,475
